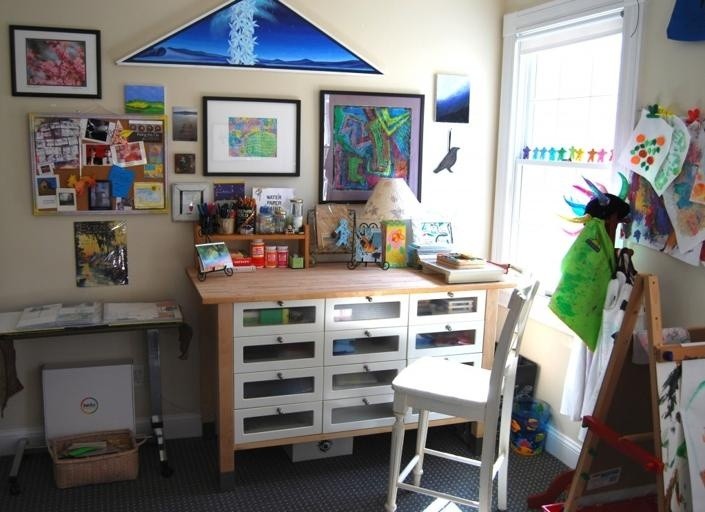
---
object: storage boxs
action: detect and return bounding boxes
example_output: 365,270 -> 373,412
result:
282,431 -> 356,462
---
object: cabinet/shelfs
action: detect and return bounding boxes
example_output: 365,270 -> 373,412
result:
183,258 -> 519,492
194,223 -> 311,273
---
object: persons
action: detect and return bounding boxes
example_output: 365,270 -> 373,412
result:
115,144 -> 128,164
86,144 -> 103,166
125,145 -> 142,162
102,146 -> 114,166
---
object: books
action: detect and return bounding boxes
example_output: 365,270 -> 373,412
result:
15,301 -> 159,329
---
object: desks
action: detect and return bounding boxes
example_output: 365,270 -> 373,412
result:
0,299 -> 185,495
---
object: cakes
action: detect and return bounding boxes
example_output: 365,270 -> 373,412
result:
436,252 -> 483,269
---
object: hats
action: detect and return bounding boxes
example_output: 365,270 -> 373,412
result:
583,194 -> 630,220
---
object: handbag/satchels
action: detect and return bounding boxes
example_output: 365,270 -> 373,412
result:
548,217 -> 615,353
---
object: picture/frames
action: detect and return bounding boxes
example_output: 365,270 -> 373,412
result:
114,0 -> 385,75
204,95 -> 301,179
418,220 -> 454,248
316,87 -> 427,206
28,113 -> 170,218
433,72 -> 473,126
9,25 -> 102,100
170,182 -> 210,222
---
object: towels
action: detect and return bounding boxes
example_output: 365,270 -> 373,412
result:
557,279 -> 646,444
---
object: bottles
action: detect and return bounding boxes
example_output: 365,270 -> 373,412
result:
265,245 -> 277,268
250,239 -> 265,270
277,245 -> 289,268
258,206 -> 274,234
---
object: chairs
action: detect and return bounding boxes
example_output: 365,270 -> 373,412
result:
385,277 -> 542,511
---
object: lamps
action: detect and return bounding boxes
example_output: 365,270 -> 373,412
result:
363,177 -> 424,269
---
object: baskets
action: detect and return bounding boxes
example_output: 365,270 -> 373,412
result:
47,428 -> 147,490
